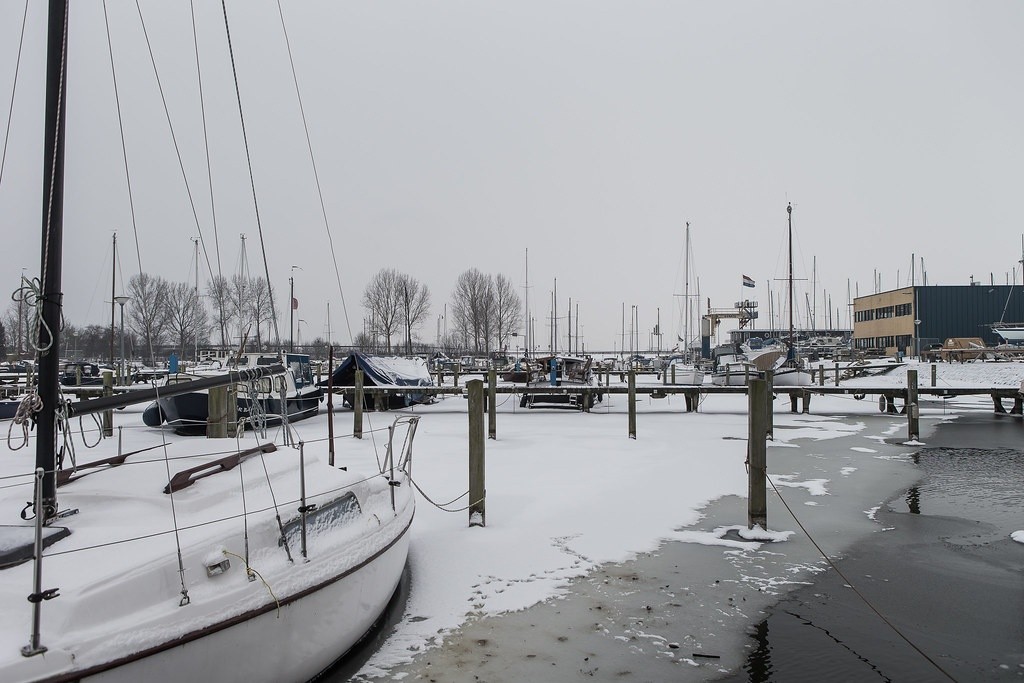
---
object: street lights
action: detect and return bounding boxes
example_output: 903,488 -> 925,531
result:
914,320 -> 921,362
114,296 -> 130,386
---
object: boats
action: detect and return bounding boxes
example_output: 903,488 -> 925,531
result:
315,352 -> 435,412
519,354 -> 603,410
61,361 -> 134,385
156,276 -> 325,436
428,248 -> 542,384
711,355 -> 758,386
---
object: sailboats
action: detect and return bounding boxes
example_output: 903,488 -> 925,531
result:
774,202 -> 811,386
662,222 -> 705,386
0,0 -> 417,683
990,233 -> 1024,344
592,302 -> 672,372
740,255 -> 849,351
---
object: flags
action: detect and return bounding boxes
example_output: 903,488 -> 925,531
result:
743,275 -> 755,288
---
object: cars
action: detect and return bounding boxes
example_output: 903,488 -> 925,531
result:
922,343 -> 943,362
